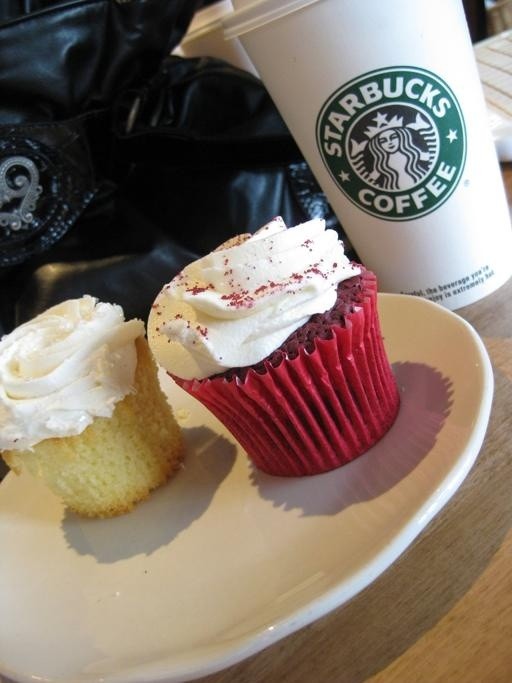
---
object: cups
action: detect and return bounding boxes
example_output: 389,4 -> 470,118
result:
223,0 -> 511,311
180,0 -> 262,82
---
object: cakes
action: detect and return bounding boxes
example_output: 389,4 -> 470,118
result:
148,217 -> 400,476
0,295 -> 182,517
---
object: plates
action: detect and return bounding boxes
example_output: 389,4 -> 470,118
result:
1,294 -> 493,683
